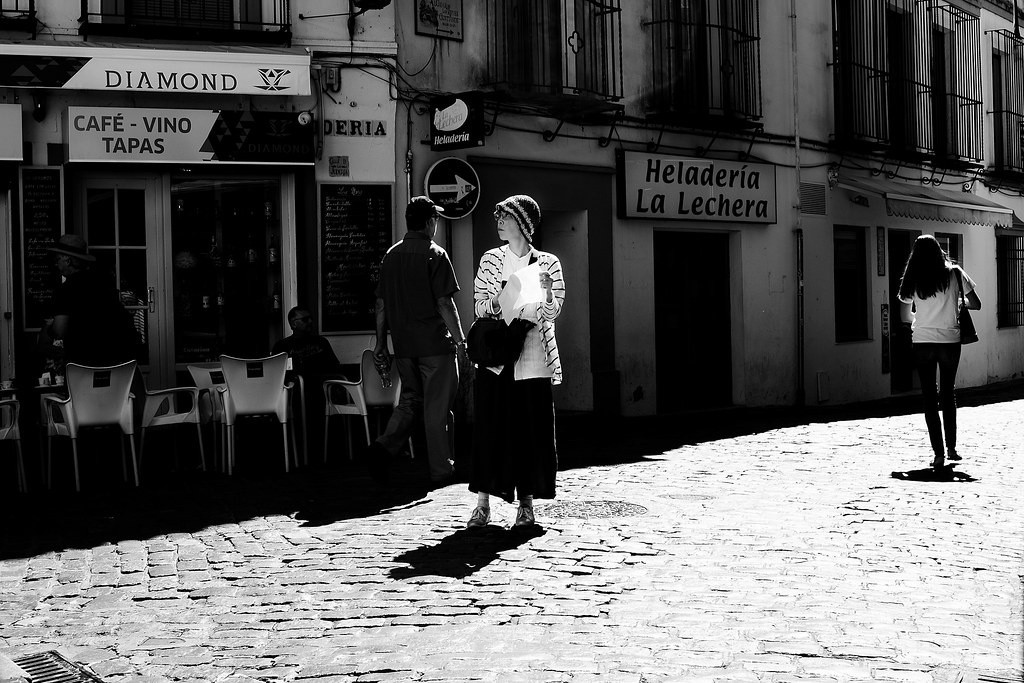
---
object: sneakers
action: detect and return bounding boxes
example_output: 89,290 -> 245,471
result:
515,506 -> 535,525
467,506 -> 492,526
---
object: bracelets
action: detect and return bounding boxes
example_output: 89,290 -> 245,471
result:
457,340 -> 466,346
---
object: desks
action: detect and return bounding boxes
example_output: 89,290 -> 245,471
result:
0,381 -> 63,469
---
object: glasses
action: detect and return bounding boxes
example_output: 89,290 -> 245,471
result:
294,313 -> 314,323
494,211 -> 513,220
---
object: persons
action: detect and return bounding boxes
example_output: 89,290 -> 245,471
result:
45,234 -> 130,365
272,306 -> 342,398
897,235 -> 982,467
463,195 -> 565,529
366,195 -> 468,480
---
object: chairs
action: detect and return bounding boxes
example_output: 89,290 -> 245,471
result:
0,349 -> 416,497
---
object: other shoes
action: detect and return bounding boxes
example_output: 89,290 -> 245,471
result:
367,445 -> 385,483
430,469 -> 457,483
947,449 -> 962,460
933,458 -> 944,470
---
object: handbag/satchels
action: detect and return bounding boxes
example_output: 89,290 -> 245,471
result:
466,316 -> 537,367
952,267 -> 979,345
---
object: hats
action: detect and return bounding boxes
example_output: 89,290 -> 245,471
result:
47,234 -> 96,261
405,196 -> 445,219
495,195 -> 542,243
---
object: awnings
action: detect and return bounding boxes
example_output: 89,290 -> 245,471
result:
839,177 -> 1014,228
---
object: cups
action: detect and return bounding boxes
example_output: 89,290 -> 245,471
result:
39,378 -> 48,385
55,375 -> 64,385
0,380 -> 12,389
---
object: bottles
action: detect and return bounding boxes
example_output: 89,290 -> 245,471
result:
264,201 -> 273,219
227,248 -> 278,267
274,294 -> 281,308
202,293 -> 225,306
